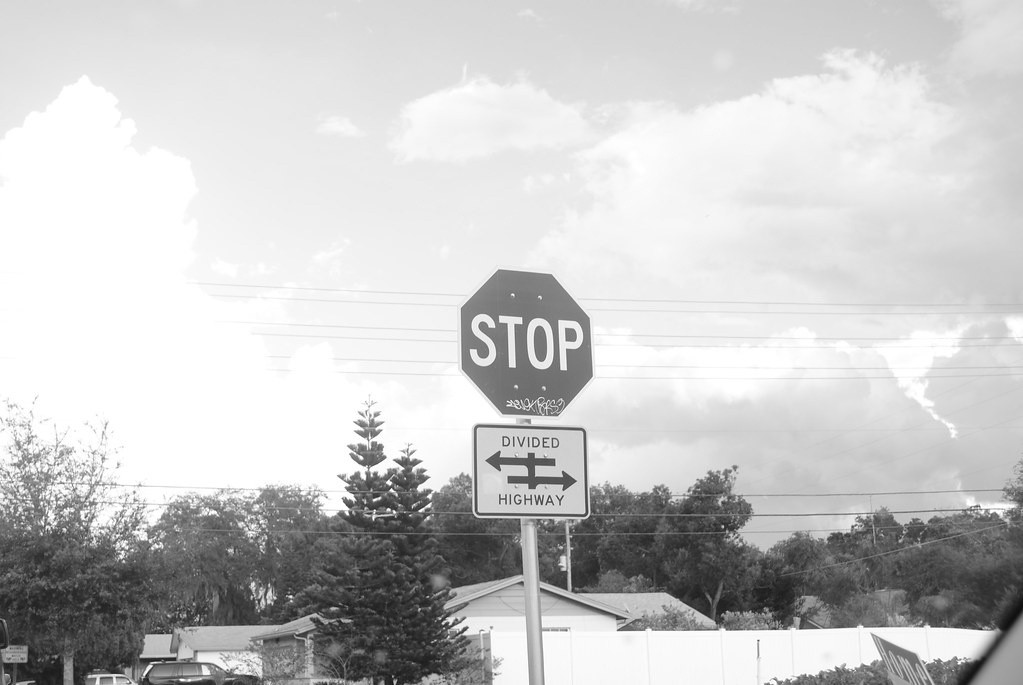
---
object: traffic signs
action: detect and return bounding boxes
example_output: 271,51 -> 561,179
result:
474,422 -> 590,518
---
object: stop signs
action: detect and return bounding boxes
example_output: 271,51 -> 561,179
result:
461,269 -> 593,418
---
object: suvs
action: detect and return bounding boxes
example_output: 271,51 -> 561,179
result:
137,659 -> 263,685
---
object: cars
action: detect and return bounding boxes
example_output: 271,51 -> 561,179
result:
78,673 -> 138,685
